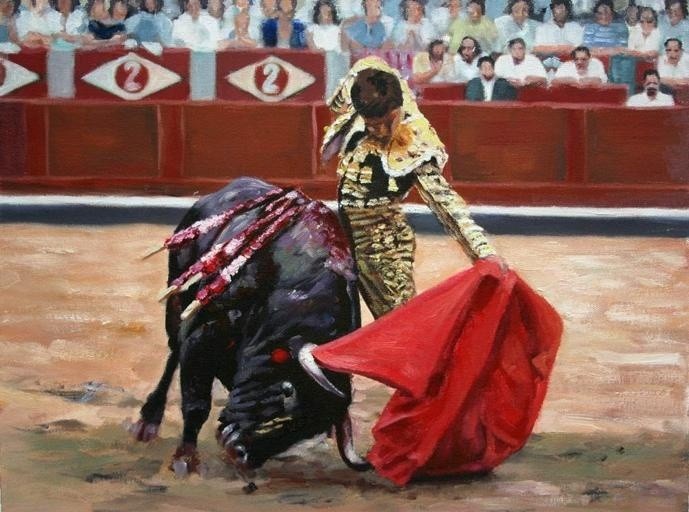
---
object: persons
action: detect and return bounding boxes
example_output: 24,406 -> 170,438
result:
319,56 -> 508,322
1,1 -> 689,108
